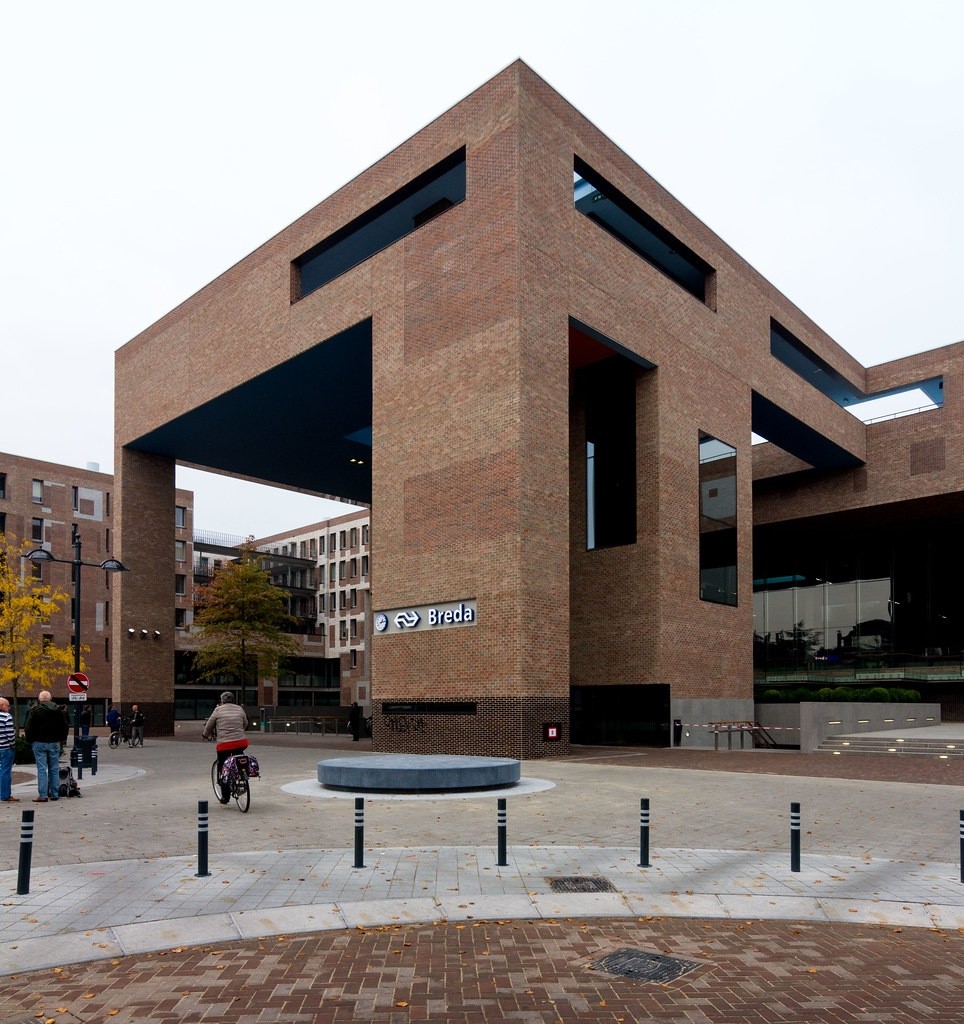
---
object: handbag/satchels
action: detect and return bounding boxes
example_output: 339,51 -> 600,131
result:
220,754 -> 259,783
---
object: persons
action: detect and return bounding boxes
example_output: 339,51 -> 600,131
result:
348,701 -> 361,742
118,705 -> 144,748
24,702 -> 36,728
106,705 -> 122,748
203,692 -> 249,803
0,698 -> 20,803
59,704 -> 71,747
25,691 -> 67,803
80,705 -> 90,742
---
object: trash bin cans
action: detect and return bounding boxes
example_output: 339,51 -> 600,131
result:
75,735 -> 98,768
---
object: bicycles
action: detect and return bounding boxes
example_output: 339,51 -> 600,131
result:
109,717 -> 143,749
204,736 -> 252,814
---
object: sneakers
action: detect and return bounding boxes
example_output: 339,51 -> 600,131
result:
51,797 -> 59,801
138,744 -> 143,747
32,797 -> 48,802
7,795 -> 20,801
129,745 -> 135,748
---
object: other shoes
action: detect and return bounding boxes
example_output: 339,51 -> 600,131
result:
220,798 -> 228,804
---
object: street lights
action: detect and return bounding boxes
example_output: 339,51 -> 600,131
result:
22,521 -> 130,743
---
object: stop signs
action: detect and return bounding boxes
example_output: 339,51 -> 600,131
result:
66,672 -> 90,693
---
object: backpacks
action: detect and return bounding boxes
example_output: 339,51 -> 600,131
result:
59,766 -> 81,797
